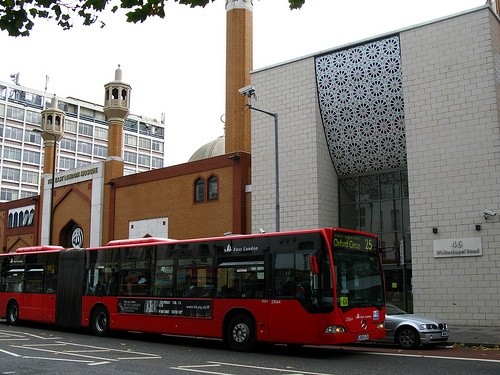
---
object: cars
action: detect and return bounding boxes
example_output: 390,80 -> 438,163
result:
375,302 -> 449,350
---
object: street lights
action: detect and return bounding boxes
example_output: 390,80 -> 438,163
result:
243,103 -> 282,232
31,128 -> 56,246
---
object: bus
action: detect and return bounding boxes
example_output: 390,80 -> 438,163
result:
0,227 -> 401,352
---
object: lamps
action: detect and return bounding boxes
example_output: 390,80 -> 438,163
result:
228,155 -> 240,161
29,197 -> 39,202
106,181 -> 116,187
